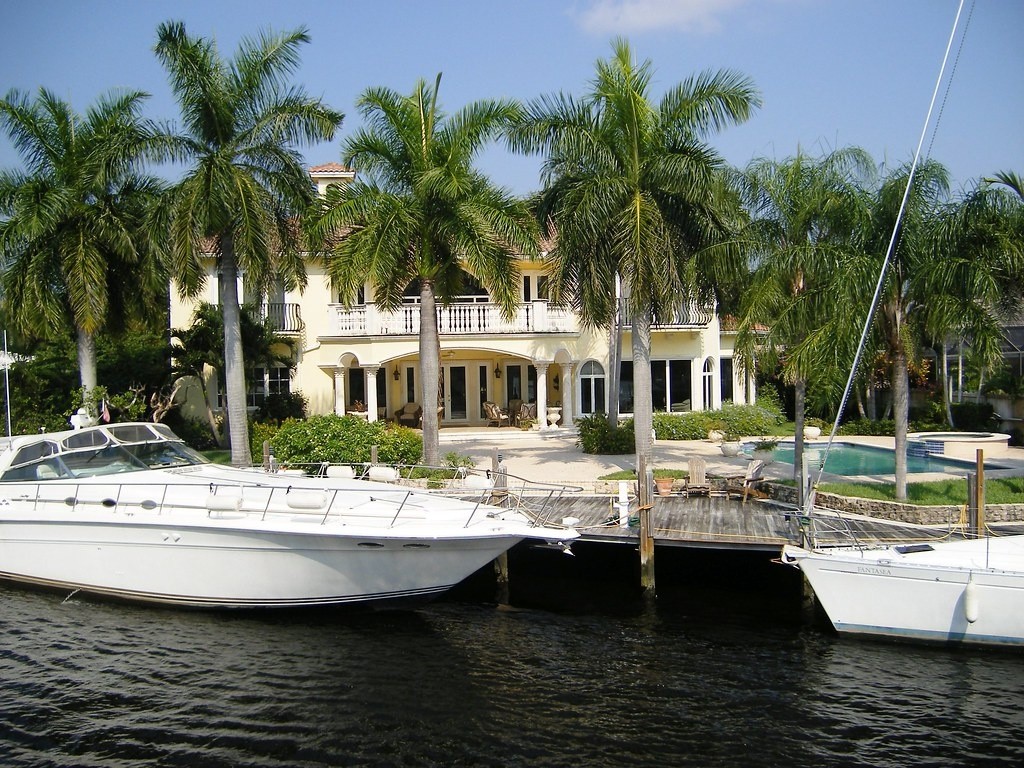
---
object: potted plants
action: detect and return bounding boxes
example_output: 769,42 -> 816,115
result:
719,432 -> 742,455
804,417 -> 824,440
752,436 -> 779,466
708,421 -> 728,442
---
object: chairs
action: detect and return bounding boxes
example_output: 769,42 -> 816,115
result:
482,399 -> 535,428
377,407 -> 386,421
724,460 -> 766,502
419,407 -> 445,430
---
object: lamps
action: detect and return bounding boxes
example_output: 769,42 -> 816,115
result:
552,374 -> 559,390
392,366 -> 401,380
493,364 -> 501,378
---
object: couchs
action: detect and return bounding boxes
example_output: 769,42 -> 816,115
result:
685,461 -> 711,498
671,399 -> 690,412
394,402 -> 422,428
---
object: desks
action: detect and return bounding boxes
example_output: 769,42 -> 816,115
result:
347,411 -> 368,421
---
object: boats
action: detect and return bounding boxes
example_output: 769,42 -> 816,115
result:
0,331 -> 581,609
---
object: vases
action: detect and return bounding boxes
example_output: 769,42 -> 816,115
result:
655,478 -> 674,495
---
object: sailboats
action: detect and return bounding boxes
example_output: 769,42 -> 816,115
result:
768,1 -> 1024,650
